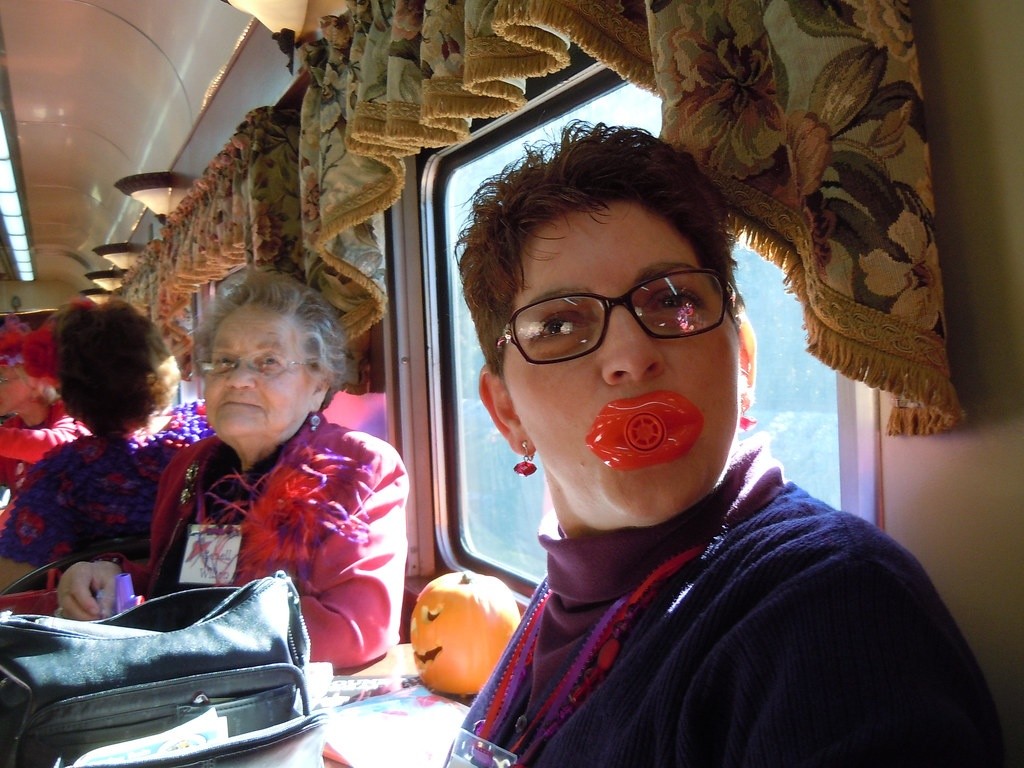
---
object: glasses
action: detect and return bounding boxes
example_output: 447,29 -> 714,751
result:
497,266 -> 738,365
196,351 -> 320,379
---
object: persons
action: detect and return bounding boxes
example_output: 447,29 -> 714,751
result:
442,119 -> 1006,768
57,272 -> 409,668
0,299 -> 218,569
0,313 -> 93,486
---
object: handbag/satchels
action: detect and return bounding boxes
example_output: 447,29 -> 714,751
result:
1,567 -> 322,768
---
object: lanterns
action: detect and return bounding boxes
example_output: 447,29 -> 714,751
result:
410,571 -> 520,694
